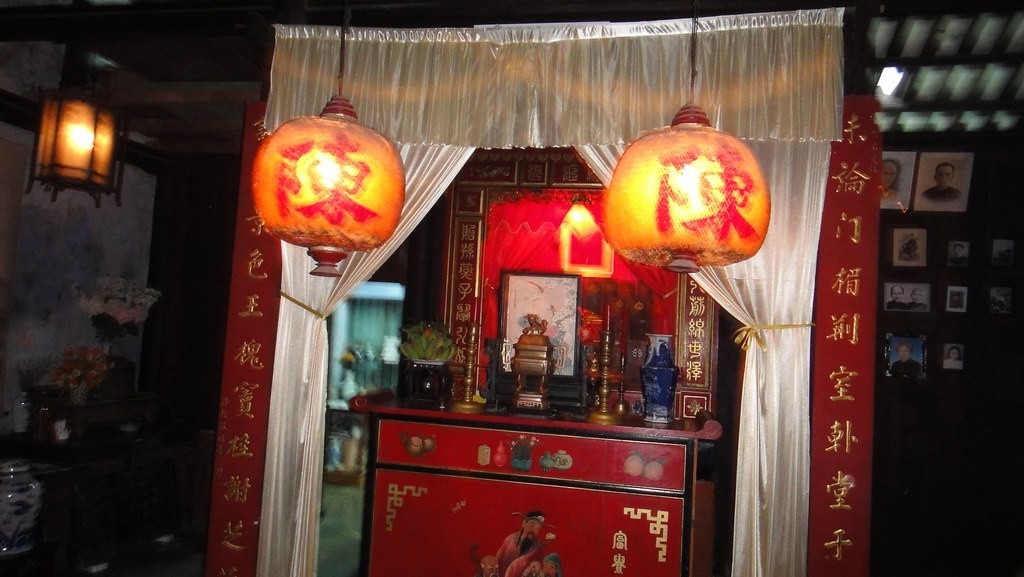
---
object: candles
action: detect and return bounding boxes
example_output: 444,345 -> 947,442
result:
472,297 -> 480,321
606,303 -> 613,332
620,310 -> 630,355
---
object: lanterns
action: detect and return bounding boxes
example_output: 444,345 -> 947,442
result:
24,92 -> 137,208
251,95 -> 406,278
605,104 -> 770,274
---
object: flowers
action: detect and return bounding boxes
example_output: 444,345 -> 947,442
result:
50,274 -> 162,391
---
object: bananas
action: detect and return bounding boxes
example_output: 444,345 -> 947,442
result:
400,327 -> 456,362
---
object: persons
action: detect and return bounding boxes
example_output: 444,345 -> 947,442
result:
880,159 -> 964,378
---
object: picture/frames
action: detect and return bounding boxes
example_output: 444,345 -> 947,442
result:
887,224 -> 930,269
988,284 -> 1014,317
886,332 -> 931,380
939,340 -> 966,374
943,281 -> 970,317
942,237 -> 972,270
989,235 -> 1018,268
879,148 -> 980,214
880,279 -> 934,316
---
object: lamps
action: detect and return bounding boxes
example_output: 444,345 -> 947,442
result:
24,45 -> 132,208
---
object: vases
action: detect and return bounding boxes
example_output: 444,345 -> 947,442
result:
12,390 -> 32,433
641,332 -> 679,425
101,355 -> 137,397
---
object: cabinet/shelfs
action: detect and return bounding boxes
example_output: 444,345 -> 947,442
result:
31,392 -> 217,577
